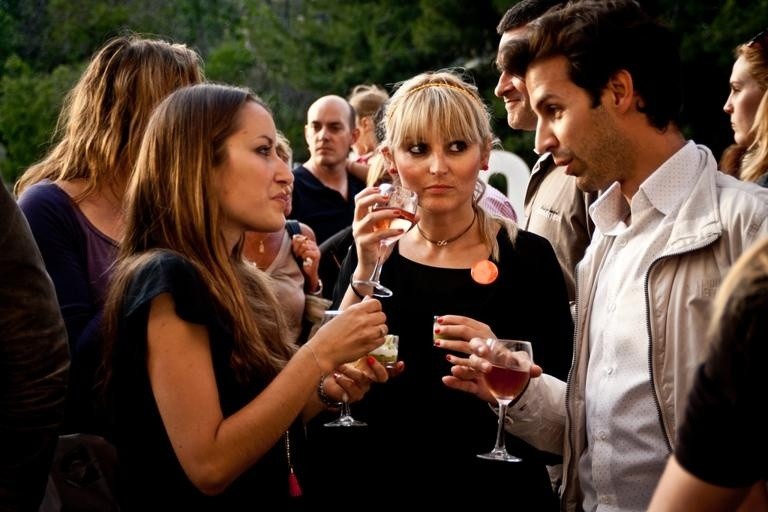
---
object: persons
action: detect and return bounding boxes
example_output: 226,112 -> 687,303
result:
441,0 -> 768,512
290,95 -> 367,223
0,182 -> 69,512
235,133 -> 318,352
648,234 -> 767,512
16,38 -> 207,422
348,84 -> 394,156
495,0 -> 598,302
334,72 -> 573,512
720,34 -> 768,186
103,84 -> 387,511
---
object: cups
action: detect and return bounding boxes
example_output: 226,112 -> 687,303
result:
324,310 -> 368,427
477,336 -> 533,463
352,182 -> 419,298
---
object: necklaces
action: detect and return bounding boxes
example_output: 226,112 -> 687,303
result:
414,209 -> 484,246
243,232 -> 280,254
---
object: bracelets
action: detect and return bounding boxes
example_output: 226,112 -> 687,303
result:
316,383 -> 345,408
